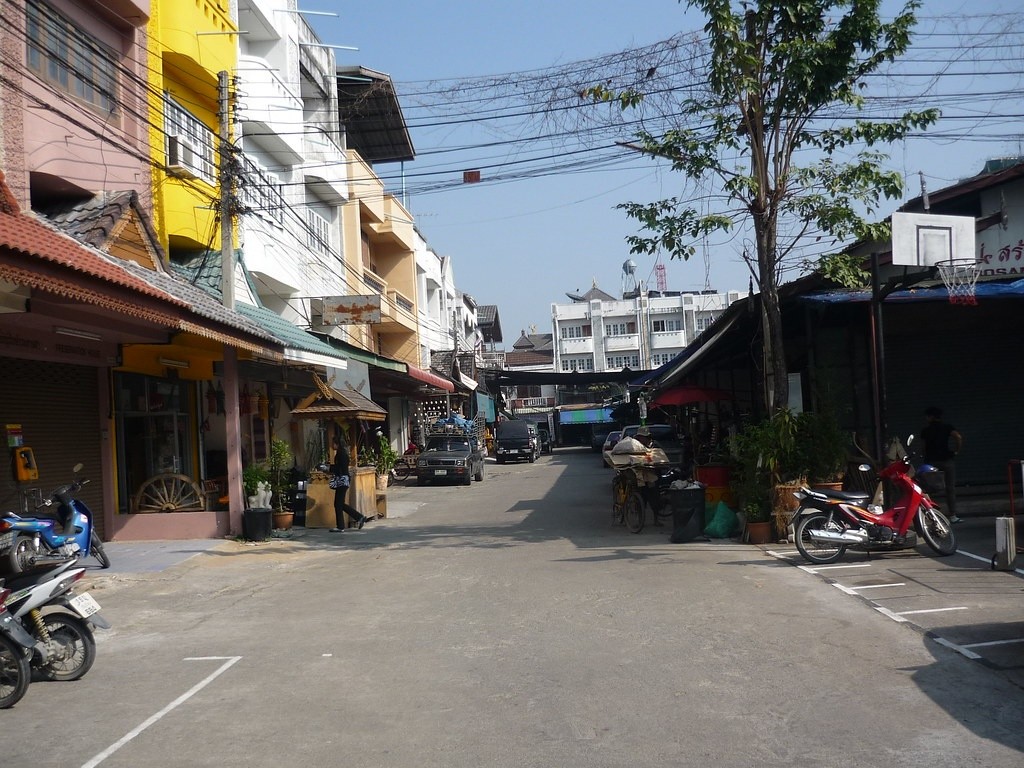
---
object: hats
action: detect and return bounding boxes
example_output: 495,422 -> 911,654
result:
635,426 -> 651,437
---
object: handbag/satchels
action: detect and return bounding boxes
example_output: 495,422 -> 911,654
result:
654,466 -> 684,496
329,472 -> 349,489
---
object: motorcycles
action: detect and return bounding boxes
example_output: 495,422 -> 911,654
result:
0,463 -> 111,576
786,434 -> 956,564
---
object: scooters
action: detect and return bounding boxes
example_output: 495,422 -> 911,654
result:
0,558 -> 113,681
0,578 -> 37,709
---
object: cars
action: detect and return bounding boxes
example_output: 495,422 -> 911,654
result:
602,431 -> 622,468
492,419 -> 553,464
592,433 -> 608,446
611,425 -> 695,488
415,435 -> 485,485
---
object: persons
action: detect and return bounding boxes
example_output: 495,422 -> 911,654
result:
323,437 -> 366,532
635,426 -> 664,528
921,406 -> 966,523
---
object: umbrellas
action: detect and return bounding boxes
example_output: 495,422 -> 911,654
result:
654,386 -> 731,460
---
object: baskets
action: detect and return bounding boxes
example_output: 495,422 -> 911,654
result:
912,471 -> 945,494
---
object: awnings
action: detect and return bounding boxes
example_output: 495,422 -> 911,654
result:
560,408 -> 616,424
290,386 -> 387,422
307,330 -> 455,392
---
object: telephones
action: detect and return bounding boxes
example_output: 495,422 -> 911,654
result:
12,447 -> 38,481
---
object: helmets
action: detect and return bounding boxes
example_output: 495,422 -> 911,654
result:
917,465 -> 938,473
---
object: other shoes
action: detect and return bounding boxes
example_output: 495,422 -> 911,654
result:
359,516 -> 366,529
655,519 -> 663,527
950,515 -> 960,522
329,528 -> 342,532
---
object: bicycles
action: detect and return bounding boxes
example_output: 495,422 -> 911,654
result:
374,450 -> 411,481
359,458 -> 394,487
649,453 -> 723,518
612,463 -> 646,534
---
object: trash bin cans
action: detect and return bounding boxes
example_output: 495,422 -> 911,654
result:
665,487 -> 708,536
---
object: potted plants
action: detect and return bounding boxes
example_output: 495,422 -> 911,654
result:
241,466 -> 272,540
766,411 -> 812,509
743,505 -> 773,544
271,438 -> 293,531
807,411 -> 852,491
361,435 -> 396,490
